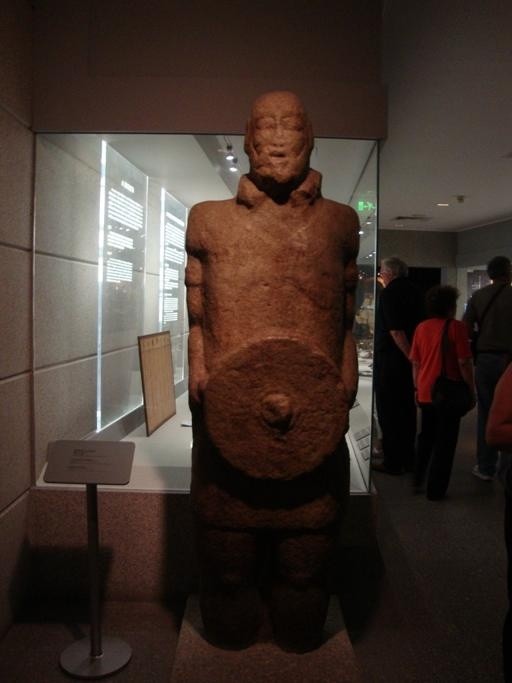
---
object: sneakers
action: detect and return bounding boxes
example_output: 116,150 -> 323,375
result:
383,456 -> 494,500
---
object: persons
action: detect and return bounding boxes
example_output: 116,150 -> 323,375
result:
182,90 -> 361,654
373,256 -> 512,500
484,362 -> 512,683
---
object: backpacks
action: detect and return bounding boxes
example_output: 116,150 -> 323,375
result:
431,374 -> 476,420
